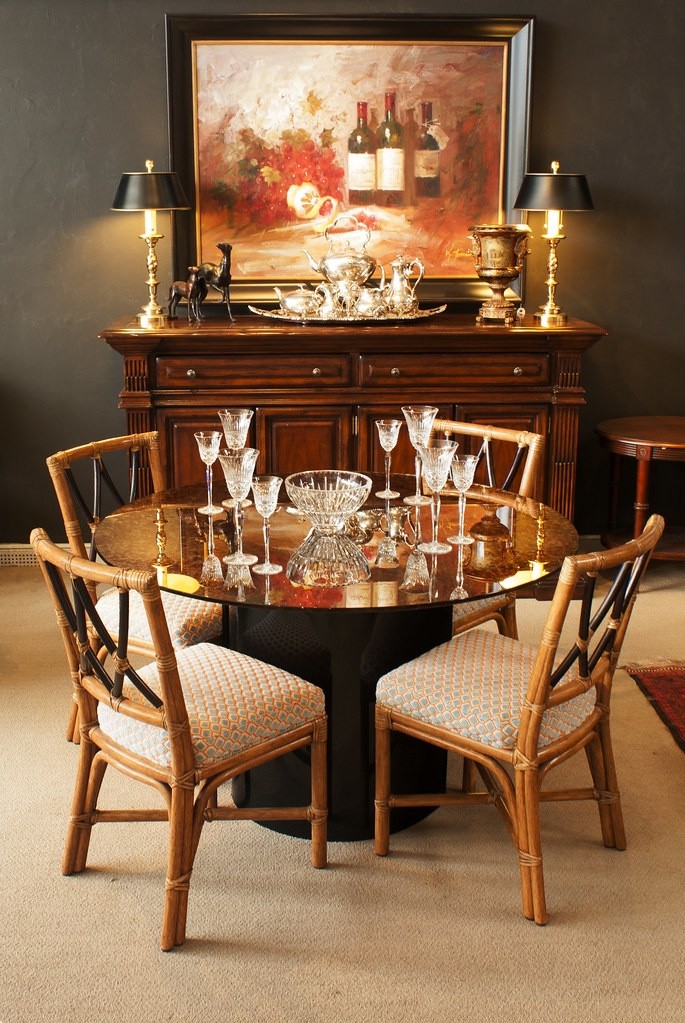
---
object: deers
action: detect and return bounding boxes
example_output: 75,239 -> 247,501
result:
198,243 -> 237,322
168,266 -> 199,323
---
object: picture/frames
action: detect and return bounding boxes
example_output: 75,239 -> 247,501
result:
163,12 -> 536,300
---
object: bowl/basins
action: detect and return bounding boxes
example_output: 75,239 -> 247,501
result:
285,527 -> 372,589
285,471 -> 373,532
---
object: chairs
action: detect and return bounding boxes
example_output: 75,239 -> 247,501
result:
47,429 -> 224,746
29,527 -> 326,952
374,512 -> 666,926
416,414 -> 544,642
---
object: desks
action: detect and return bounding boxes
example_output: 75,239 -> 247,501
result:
92,470 -> 574,843
592,416 -> 685,564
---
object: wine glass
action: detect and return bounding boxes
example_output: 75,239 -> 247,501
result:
198,517 -> 256,596
447,455 -> 480,545
218,409 -> 255,508
193,431 -> 225,514
215,448 -> 260,565
467,223 -> 532,324
414,439 -> 453,553
251,476 -> 283,576
401,405 -> 440,507
375,420 -> 401,499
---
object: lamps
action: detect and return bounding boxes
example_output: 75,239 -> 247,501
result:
109,160 -> 193,328
513,160 -> 596,327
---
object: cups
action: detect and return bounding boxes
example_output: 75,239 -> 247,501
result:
462,501 -> 519,583
375,506 -> 469,600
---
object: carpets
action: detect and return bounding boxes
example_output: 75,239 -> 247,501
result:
615,659 -> 685,751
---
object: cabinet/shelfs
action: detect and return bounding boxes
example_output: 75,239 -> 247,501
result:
94,311 -> 601,519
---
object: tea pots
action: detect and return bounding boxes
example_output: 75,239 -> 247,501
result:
300,214 -> 377,287
374,254 -> 425,315
273,283 -> 394,318
